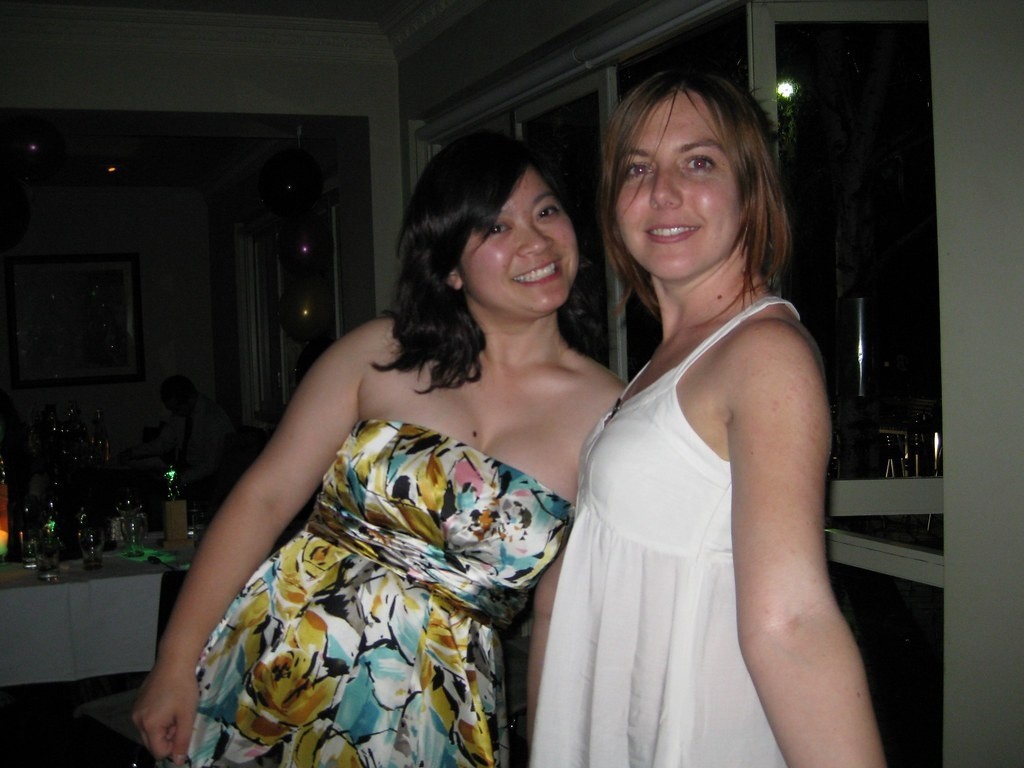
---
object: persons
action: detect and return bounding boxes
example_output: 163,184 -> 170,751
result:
524,58 -> 885,767
129,133 -> 631,768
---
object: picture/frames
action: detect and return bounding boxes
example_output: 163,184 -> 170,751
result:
5,252 -> 148,389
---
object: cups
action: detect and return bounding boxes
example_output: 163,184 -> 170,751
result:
119,513 -> 148,556
34,537 -> 59,581
22,530 -> 43,570
78,516 -> 107,571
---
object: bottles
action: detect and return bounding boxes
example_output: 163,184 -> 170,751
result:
191,501 -> 206,548
91,409 -> 105,463
828,407 -> 840,479
104,428 -> 109,461
163,464 -> 186,540
882,413 -> 935,478
0,455 -> 10,563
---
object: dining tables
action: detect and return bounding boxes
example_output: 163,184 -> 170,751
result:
1,522 -> 214,690
